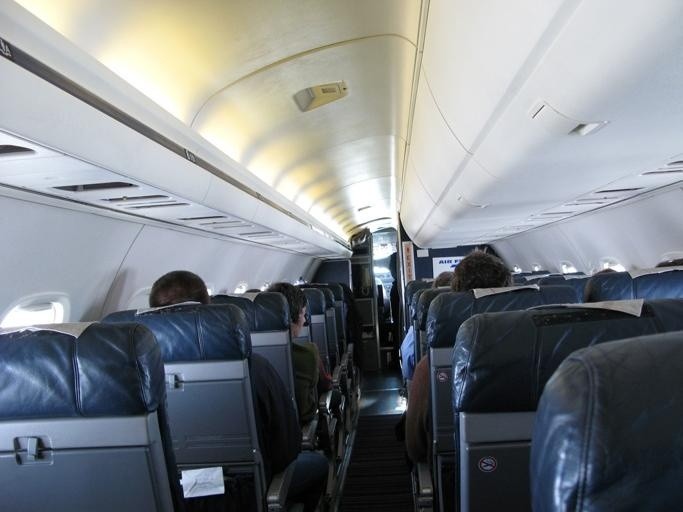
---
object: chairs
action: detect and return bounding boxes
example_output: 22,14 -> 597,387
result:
377,300 -> 394,363
396,272 -> 682,510
1,272 -> 361,509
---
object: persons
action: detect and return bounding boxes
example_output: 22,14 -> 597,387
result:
149,270 -> 330,512
399,270 -> 455,393
388,242 -> 398,364
405,251 -> 514,463
265,282 -> 330,449
